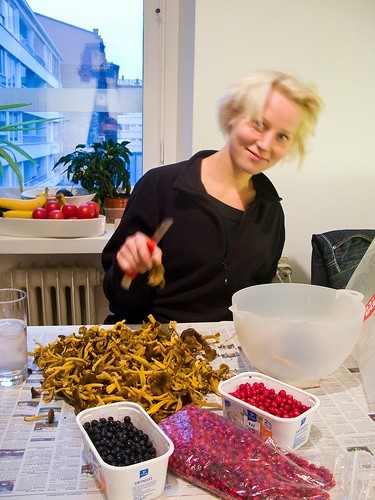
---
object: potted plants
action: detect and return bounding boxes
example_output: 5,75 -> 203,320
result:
52,141 -> 133,224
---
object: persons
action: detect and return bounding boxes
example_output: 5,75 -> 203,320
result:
101,71 -> 325,326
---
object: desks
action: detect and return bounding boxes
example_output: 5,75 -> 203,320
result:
0,222 -> 116,254
2,320 -> 375,500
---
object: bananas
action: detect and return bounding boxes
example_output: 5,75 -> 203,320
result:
54,193 -> 68,211
0,187 -> 49,219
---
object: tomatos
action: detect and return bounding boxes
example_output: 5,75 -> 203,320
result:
32,200 -> 100,219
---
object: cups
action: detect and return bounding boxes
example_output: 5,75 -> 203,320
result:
0,288 -> 27,387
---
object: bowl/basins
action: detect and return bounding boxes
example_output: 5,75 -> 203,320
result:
228,283 -> 365,388
21,186 -> 99,207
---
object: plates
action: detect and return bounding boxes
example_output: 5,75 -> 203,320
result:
1,214 -> 106,238
